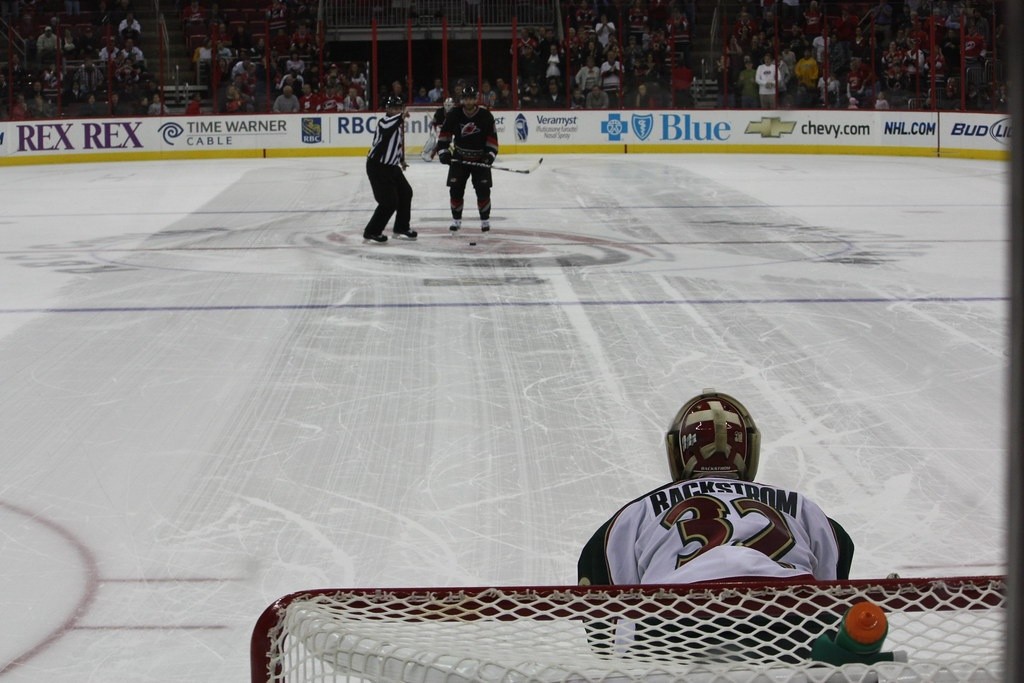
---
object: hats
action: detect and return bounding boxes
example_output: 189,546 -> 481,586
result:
44,26 -> 51,31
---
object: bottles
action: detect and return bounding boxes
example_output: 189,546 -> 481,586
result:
835,602 -> 888,656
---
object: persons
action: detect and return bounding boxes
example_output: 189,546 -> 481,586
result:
420,98 -> 456,162
435,83 -> 498,238
576,388 -> 855,671
0,0 -> 1012,122
362,96 -> 418,245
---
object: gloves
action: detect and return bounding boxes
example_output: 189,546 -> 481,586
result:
478,151 -> 496,165
437,147 -> 451,165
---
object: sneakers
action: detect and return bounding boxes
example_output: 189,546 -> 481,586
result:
362,229 -> 388,245
450,220 -> 462,236
481,220 -> 490,236
392,229 -> 418,241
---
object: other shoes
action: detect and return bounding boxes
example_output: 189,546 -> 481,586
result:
421,152 -> 432,162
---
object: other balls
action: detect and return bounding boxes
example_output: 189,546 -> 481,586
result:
470,242 -> 476,246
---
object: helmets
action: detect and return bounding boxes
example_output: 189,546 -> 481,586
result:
462,84 -> 478,101
666,392 -> 761,483
385,96 -> 403,112
444,98 -> 455,105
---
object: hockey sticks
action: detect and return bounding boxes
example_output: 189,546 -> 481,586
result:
450,157 -> 543,174
425,110 -> 454,153
